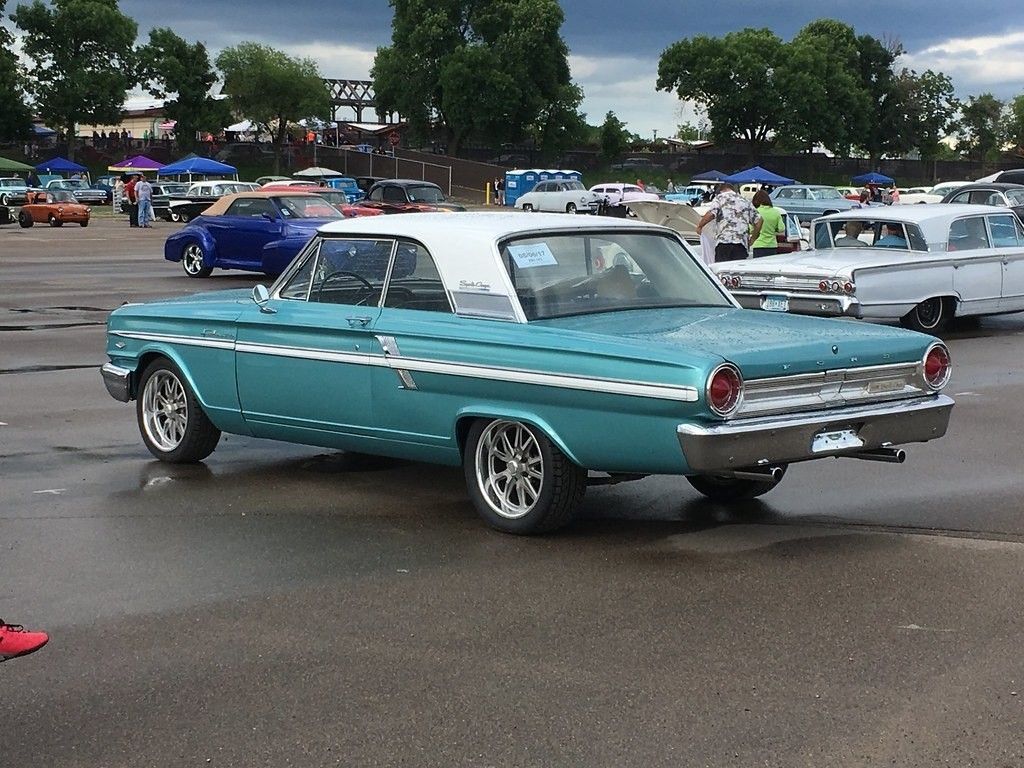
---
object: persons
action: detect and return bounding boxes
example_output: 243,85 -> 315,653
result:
497,178 -> 506,206
27,176 -> 35,187
767,186 -> 773,194
0,619 -> 49,663
637,179 -> 644,189
748,190 -> 785,258
761,183 -> 768,190
80,172 -> 87,180
71,172 -> 81,185
14,173 -> 19,178
668,178 -> 674,193
24,129 -> 348,159
113,174 -> 153,228
493,177 -> 499,205
707,184 -> 713,194
697,183 -> 763,262
835,185 -> 908,249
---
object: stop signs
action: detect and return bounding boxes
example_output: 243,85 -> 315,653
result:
389,133 -> 400,147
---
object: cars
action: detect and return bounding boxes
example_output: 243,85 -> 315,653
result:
120,178 -> 467,222
36,175 -> 108,205
594,214 -> 800,280
490,154 -> 697,172
17,189 -> 91,228
339,144 -> 393,156
665,186 -> 713,203
794,181 -> 977,204
736,183 -> 762,199
0,178 -> 40,206
941,169 -> 1024,237
1,142 -> 322,167
514,180 -> 600,215
71,171 -> 144,203
768,185 -> 886,222
589,183 -> 660,217
99,211 -> 954,532
164,190 -> 417,282
709,204 -> 1024,335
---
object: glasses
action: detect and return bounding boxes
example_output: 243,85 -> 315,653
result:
140,175 -> 145,178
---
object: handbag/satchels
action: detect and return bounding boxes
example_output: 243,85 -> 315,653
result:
148,205 -> 156,222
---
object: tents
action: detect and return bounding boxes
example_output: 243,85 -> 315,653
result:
690,166 -> 795,195
35,157 -> 91,185
34,127 -> 55,135
107,155 -> 168,182
158,115 -> 339,149
0,157 -> 36,177
294,167 -> 342,178
850,173 -> 894,188
157,157 -> 238,187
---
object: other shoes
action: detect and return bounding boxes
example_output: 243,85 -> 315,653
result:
130,224 -> 133,227
139,225 -> 144,228
135,223 -> 139,227
145,224 -> 152,227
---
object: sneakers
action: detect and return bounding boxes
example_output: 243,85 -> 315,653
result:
0,617 -> 48,662
